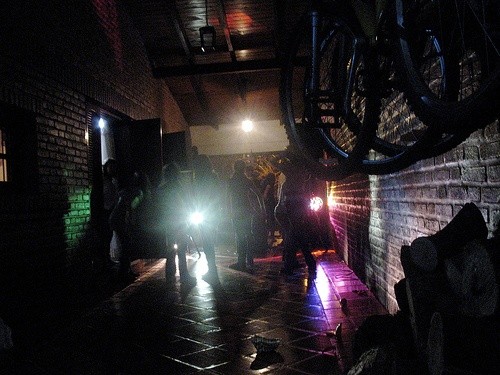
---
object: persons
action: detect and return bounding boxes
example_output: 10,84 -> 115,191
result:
110,145 -> 318,281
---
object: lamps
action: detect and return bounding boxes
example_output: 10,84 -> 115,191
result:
199,0 -> 216,52
